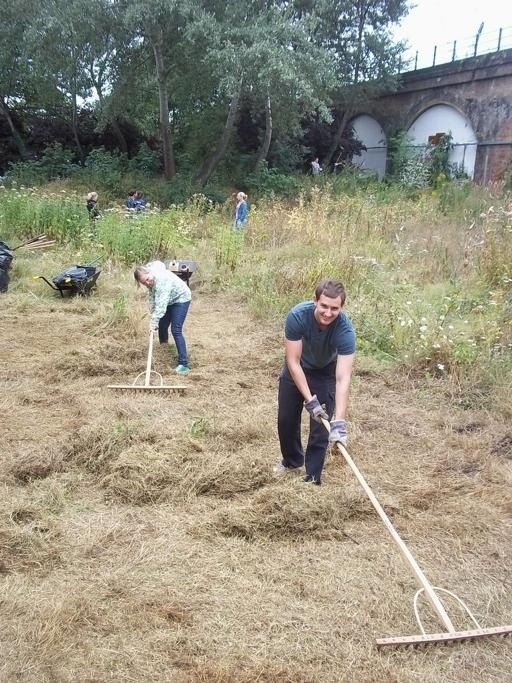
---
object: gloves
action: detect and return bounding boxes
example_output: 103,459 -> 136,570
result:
303,393 -> 329,425
332,420 -> 348,450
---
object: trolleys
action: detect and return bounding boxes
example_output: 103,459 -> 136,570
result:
32,264 -> 102,298
163,259 -> 198,287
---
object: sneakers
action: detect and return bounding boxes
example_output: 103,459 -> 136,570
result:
273,462 -> 289,475
174,365 -> 190,373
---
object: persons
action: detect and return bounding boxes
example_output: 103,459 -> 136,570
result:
127,188 -> 138,208
233,191 -> 248,227
273,278 -> 357,486
87,191 -> 101,236
135,189 -> 146,207
134,259 -> 193,372
